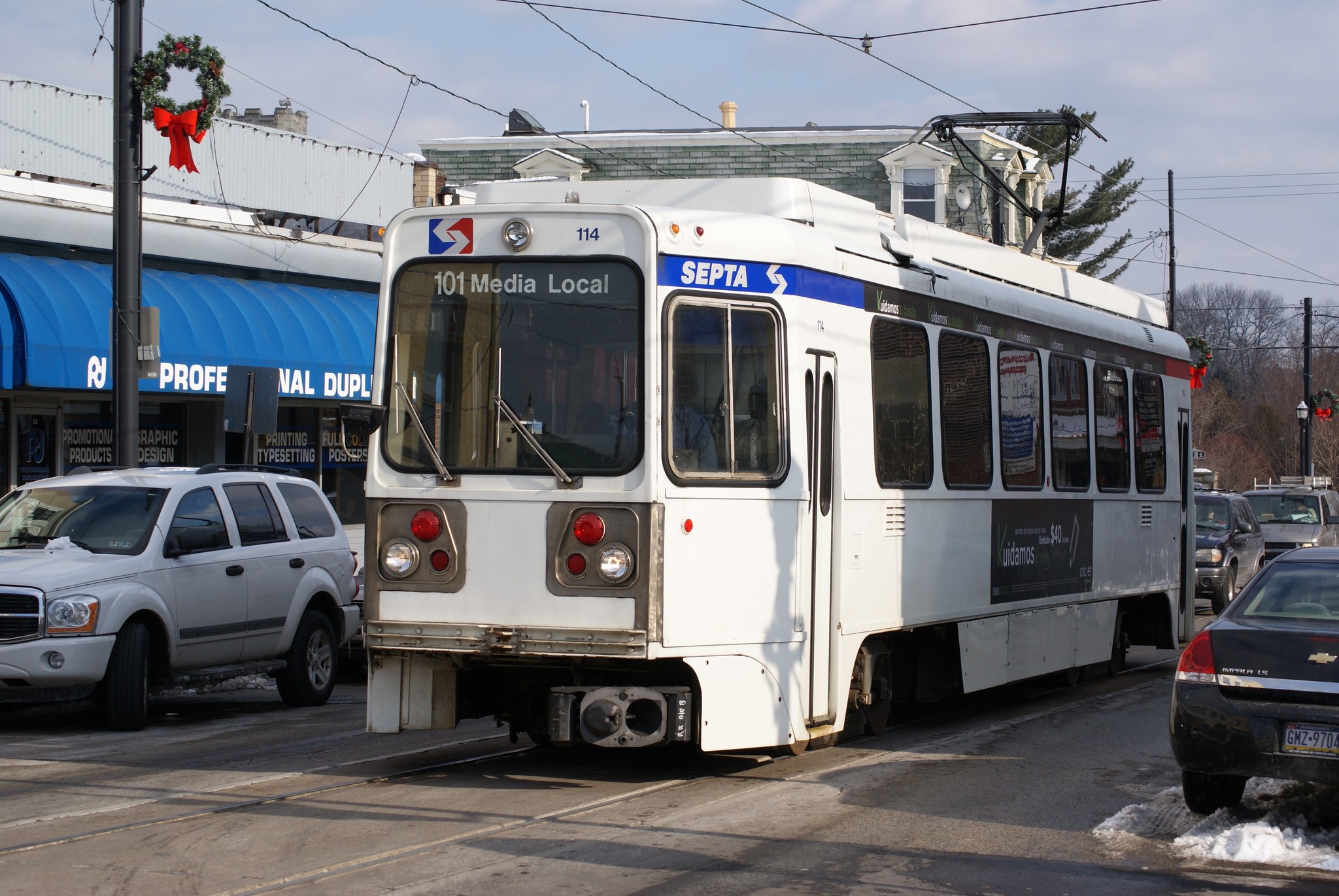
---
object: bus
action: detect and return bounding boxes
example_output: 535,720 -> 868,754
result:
357,175 -> 1204,766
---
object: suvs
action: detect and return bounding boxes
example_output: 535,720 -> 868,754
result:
0,461 -> 362,733
1237,476 -> 1339,565
1192,487 -> 1267,615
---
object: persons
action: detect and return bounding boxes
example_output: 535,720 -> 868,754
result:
610,366 -> 718,472
1281,497 -> 1316,518
1200,505 -> 1227,526
66,466 -> 92,476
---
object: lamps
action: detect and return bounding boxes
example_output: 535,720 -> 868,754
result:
509,108 -> 545,134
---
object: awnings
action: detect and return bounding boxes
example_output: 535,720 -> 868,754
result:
0,252 -> 441,402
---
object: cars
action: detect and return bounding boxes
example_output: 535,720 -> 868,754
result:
339,522 -> 369,687
1171,549 -> 1339,816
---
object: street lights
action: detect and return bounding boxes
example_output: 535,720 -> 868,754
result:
1294,401 -> 1307,477
